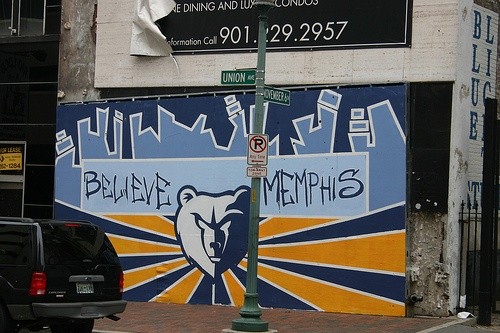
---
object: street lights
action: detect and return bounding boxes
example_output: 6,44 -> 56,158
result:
231,0 -> 276,332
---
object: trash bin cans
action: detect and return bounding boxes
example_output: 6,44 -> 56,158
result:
467,249 -> 499,306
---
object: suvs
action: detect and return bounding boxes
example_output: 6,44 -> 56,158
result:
0,215 -> 128,333
